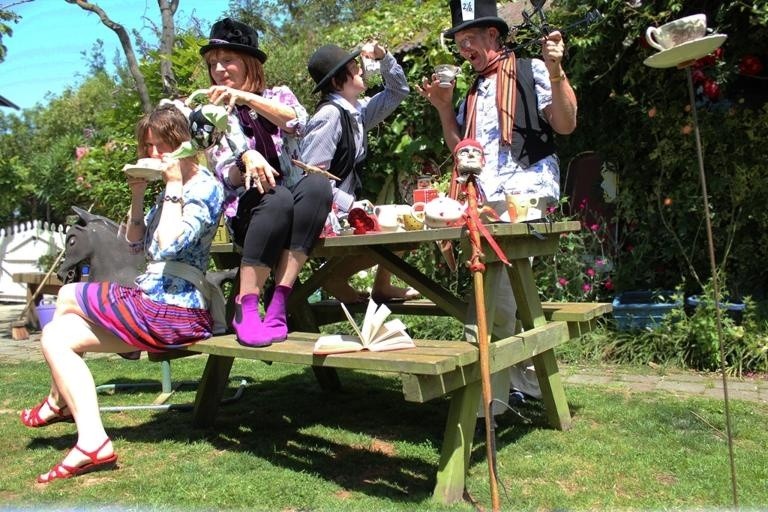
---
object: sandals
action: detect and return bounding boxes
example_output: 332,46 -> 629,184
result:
37,438 -> 118,485
19,397 -> 76,428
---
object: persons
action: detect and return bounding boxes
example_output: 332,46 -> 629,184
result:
21,101 -> 225,484
185,19 -> 339,347
292,34 -> 423,307
454,138 -> 486,174
416,1 -> 584,428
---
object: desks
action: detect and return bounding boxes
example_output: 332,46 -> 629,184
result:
10,269 -> 89,326
208,218 -> 585,505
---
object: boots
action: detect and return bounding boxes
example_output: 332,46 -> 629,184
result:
262,284 -> 293,343
231,294 -> 272,348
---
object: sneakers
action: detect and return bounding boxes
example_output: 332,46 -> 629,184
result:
509,389 -> 527,405
473,418 -> 498,441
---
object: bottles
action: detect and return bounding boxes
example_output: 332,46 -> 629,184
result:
413,176 -> 438,211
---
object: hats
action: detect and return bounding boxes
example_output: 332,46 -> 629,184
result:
439,0 -> 508,55
308,43 -> 362,94
199,17 -> 267,64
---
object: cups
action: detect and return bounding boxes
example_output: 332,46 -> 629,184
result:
137,156 -> 162,168
397,213 -> 427,231
432,65 -> 461,88
374,205 -> 403,231
504,194 -> 540,227
646,13 -> 709,52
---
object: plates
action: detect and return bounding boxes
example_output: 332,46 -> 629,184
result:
644,34 -> 728,70
124,167 -> 166,180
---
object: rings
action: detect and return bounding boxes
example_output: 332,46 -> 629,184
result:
243,172 -> 250,178
253,174 -> 260,183
259,171 -> 266,179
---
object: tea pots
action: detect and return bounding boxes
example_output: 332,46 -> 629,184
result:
413,193 -> 471,229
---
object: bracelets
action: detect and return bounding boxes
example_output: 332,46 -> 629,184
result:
548,71 -> 569,87
126,213 -> 145,228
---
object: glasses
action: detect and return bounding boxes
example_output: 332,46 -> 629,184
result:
452,32 -> 483,51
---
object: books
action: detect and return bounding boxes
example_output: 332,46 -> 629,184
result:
310,297 -> 416,356
